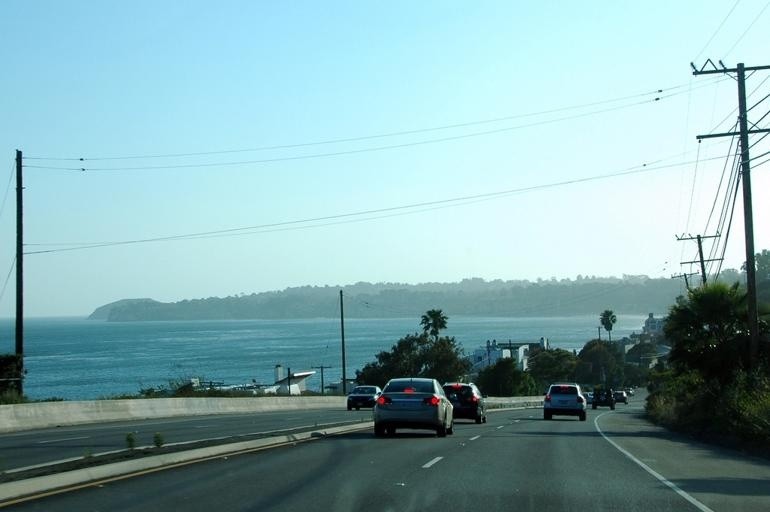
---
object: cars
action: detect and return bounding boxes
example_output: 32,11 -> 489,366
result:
582,388 -> 636,410
347,385 -> 381,411
373,374 -> 454,438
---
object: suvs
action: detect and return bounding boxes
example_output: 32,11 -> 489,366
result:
442,381 -> 488,424
543,384 -> 588,421
